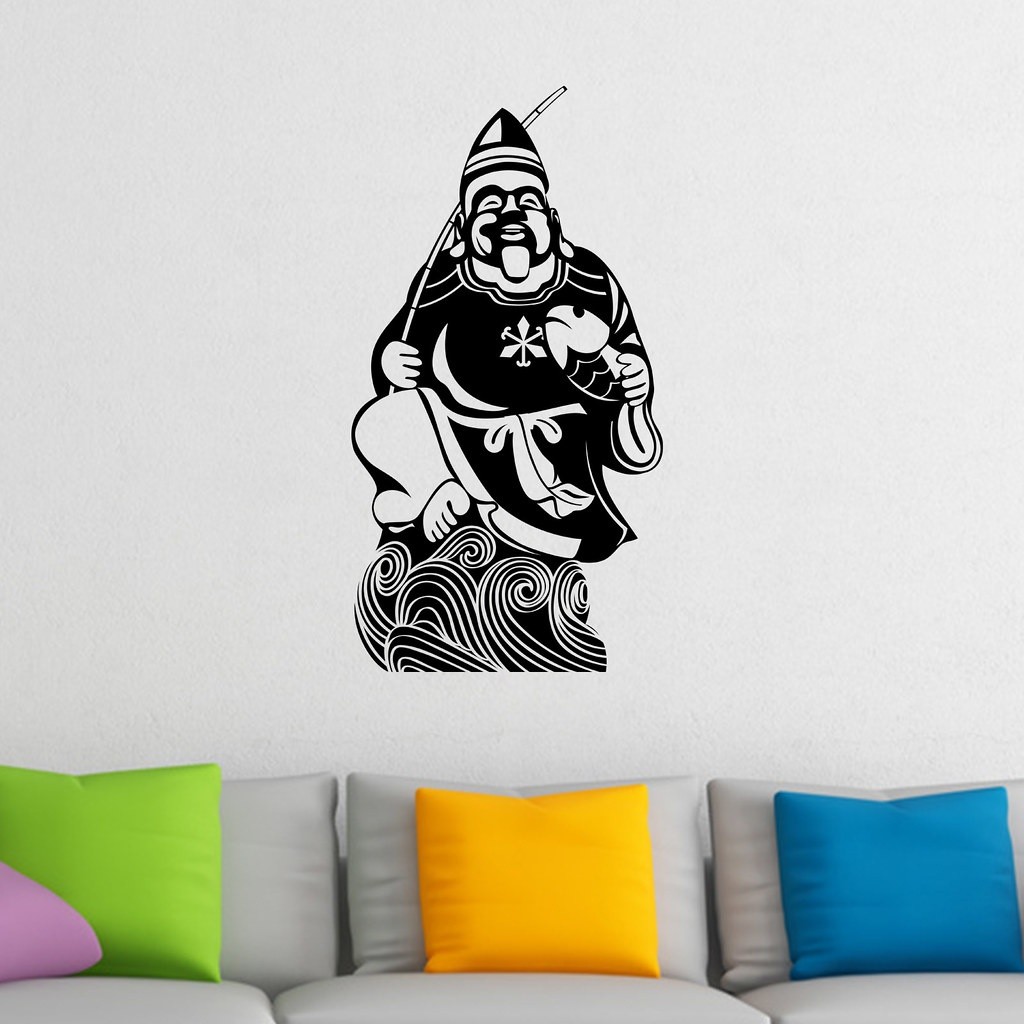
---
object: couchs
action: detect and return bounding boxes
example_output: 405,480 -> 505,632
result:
0,771 -> 1024,1024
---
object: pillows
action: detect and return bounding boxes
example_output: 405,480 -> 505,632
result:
0,861 -> 103,983
0,762 -> 226,984
413,782 -> 660,978
772,785 -> 1024,983
